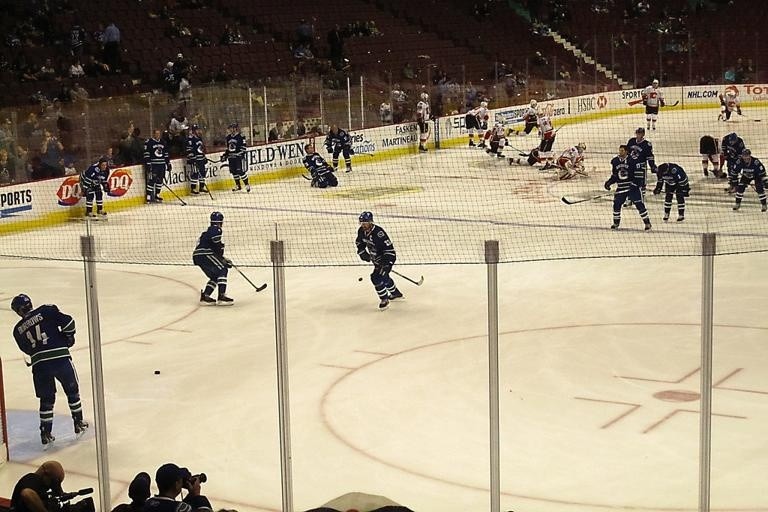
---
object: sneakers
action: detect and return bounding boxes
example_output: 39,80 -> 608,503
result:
761,204 -> 767,211
41,433 -> 55,444
508,128 -> 513,136
218,295 -> 233,301
191,189 -> 199,194
97,210 -> 107,215
677,216 -> 684,221
611,224 -> 619,229
645,223 -> 651,230
509,158 -> 513,165
74,420 -> 88,433
724,186 -> 736,193
155,193 -> 163,200
388,292 -> 402,300
146,194 -> 152,202
379,299 -> 389,308
232,184 -> 241,191
86,212 -> 96,217
199,187 -> 209,192
469,141 -> 476,146
245,184 -> 250,192
663,213 -> 669,220
497,152 -> 505,157
733,203 -> 740,210
346,167 -> 351,172
200,296 -> 216,302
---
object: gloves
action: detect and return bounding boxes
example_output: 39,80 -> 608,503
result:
328,148 -> 333,153
653,186 -> 662,194
651,165 -> 657,173
660,101 -> 664,107
605,181 -> 611,191
360,250 -> 370,262
643,101 -> 647,104
349,149 -> 354,155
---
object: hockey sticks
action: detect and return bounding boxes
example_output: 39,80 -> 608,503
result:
732,110 -> 762,122
562,193 -> 615,204
639,101 -> 679,107
302,166 -> 340,180
332,150 -> 374,157
508,123 -> 597,178
232,264 -> 267,291
157,177 -> 194,206
195,164 -> 222,201
369,257 -> 423,285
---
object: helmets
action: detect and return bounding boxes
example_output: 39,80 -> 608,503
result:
192,123 -> 201,131
653,79 -> 658,83
729,133 -> 737,141
530,99 -> 537,106
498,116 -> 506,124
421,92 -> 428,99
729,89 -> 735,96
359,211 -> 373,222
11,294 -> 31,311
228,121 -> 239,128
578,142 -> 586,150
210,212 -> 223,222
636,127 -> 645,133
480,102 -> 487,107
742,148 -> 751,155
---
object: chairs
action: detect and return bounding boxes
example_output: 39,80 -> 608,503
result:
0,0 -> 767,178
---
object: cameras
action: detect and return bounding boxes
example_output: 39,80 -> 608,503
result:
49,136 -> 58,143
180,467 -> 207,489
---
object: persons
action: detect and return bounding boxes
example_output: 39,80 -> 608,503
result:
719,89 -> 742,121
355,211 -> 404,311
653,163 -> 690,221
8,292 -> 89,444
605,145 -> 652,230
623,127 -> 657,207
642,79 -> 665,130
508,99 -> 556,166
466,102 -> 489,147
191,211 -> 234,306
2,0 -> 768,105
143,129 -> 171,204
558,142 -> 587,177
302,144 -> 338,188
483,114 -> 507,158
109,470 -> 158,511
139,464 -> 219,512
324,125 -> 352,172
700,132 -> 767,212
221,122 -> 251,192
184,123 -> 209,195
416,93 -> 431,152
10,460 -> 85,510
3,105 -> 326,185
